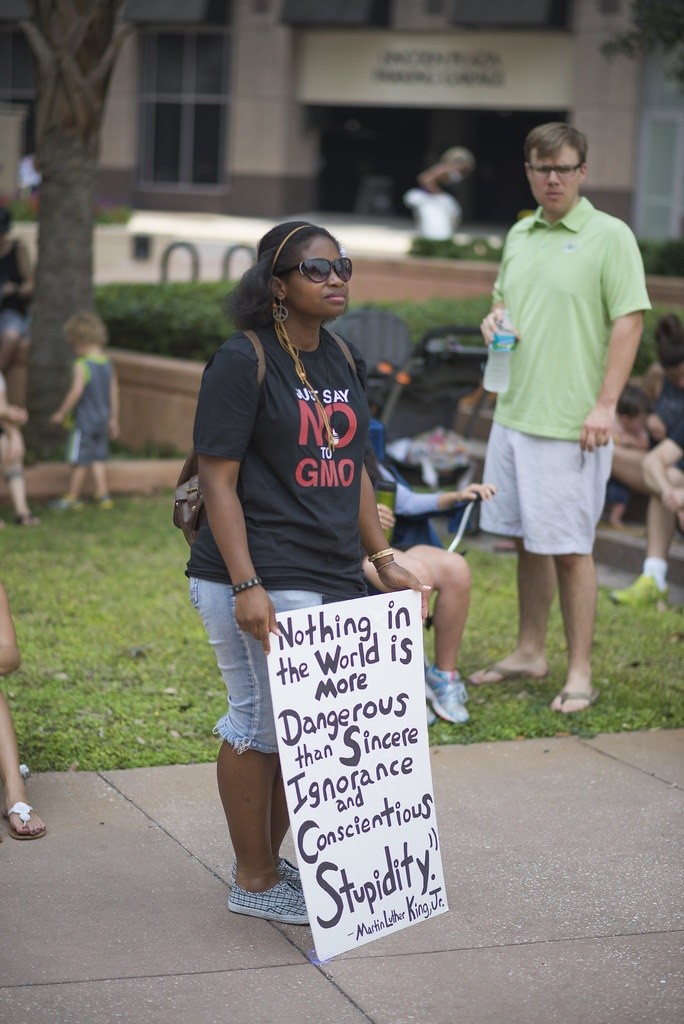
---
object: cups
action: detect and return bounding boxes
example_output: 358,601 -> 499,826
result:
374,480 -> 396,545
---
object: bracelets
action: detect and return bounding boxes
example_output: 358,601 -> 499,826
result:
377,560 -> 395,572
368,548 -> 394,562
231,577 -> 262,594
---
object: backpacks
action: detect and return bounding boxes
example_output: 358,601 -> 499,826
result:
173,330 -> 357,546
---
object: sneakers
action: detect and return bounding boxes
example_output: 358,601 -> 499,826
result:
423,698 -> 437,728
425,662 -> 470,724
610,573 -> 669,609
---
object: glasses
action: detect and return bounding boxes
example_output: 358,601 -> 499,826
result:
269,257 -> 353,283
530,159 -> 581,179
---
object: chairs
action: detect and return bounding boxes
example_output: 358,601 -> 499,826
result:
367,416 -> 484,553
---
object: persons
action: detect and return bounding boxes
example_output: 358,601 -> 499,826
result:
184,221 -> 431,925
0,204 -> 33,371
0,372 -> 43,530
606,314 -> 684,606
360,462 -> 497,726
0,582 -> 47,840
468,121 -> 652,711
48,310 -> 120,513
408,147 -> 473,239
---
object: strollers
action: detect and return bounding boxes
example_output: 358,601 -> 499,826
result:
323,309 -> 500,539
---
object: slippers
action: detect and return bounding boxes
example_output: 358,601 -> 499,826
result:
465,660 -> 550,685
549,680 -> 600,714
1,797 -> 46,840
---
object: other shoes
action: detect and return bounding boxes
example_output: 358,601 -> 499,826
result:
230,853 -> 302,888
228,874 -> 308,925
49,495 -> 84,512
89,495 -> 114,511
16,512 -> 43,527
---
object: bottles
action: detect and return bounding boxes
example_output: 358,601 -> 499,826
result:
482,308 -> 515,396
